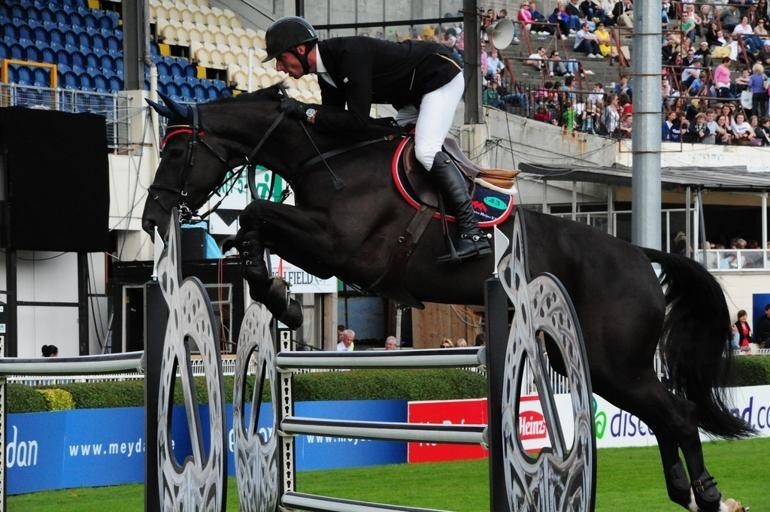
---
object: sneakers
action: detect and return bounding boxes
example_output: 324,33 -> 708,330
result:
530,28 -> 577,41
514,36 -> 521,41
595,52 -> 603,58
587,52 -> 596,58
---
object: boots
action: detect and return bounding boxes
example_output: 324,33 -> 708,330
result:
428,150 -> 493,266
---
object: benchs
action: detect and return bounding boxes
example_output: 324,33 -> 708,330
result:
479,20 -> 751,104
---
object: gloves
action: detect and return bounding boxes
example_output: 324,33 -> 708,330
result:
280,96 -> 310,122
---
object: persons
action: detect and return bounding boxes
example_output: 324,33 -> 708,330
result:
671,231 -> 770,269
455,338 -> 467,347
422,0 -> 770,147
735,310 -> 751,354
42,345 -> 58,357
475,334 -> 486,346
384,336 -> 397,349
756,305 -> 770,349
440,339 -> 453,347
338,325 -> 345,343
261,17 -> 492,267
730,324 -> 740,349
337,329 -> 355,352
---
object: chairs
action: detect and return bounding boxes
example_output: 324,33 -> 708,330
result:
0,1 -> 322,131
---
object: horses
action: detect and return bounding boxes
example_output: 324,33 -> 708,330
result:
141,80 -> 761,511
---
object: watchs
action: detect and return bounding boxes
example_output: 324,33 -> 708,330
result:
304,107 -> 317,123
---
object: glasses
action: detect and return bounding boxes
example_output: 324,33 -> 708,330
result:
521,4 -> 529,7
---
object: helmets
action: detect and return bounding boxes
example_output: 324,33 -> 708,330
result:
260,16 -> 320,64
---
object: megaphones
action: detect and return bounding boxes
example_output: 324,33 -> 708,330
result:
480,18 -> 514,50
